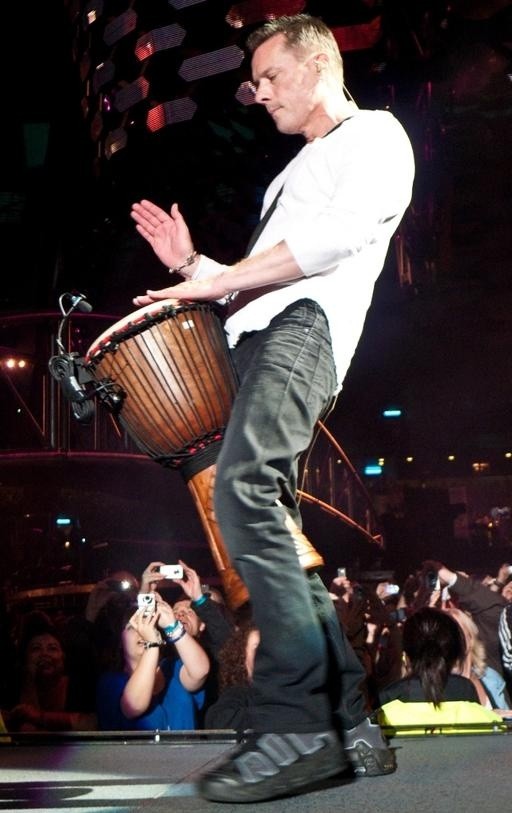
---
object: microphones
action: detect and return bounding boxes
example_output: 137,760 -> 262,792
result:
64,292 -> 92,314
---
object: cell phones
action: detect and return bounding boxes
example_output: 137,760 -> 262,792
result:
384,585 -> 400,593
109,581 -> 131,592
338,568 -> 346,577
160,565 -> 184,579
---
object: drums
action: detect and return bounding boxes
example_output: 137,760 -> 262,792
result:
87,299 -> 325,613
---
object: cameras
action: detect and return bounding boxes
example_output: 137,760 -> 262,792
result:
427,567 -> 438,586
137,594 -> 155,616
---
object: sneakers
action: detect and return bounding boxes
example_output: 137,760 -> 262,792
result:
191,711 -> 401,803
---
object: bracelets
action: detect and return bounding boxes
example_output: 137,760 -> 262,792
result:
168,250 -> 198,274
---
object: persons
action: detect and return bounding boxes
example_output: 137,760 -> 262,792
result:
327,561 -> 510,736
0,561 -> 263,741
132,13 -> 418,804
